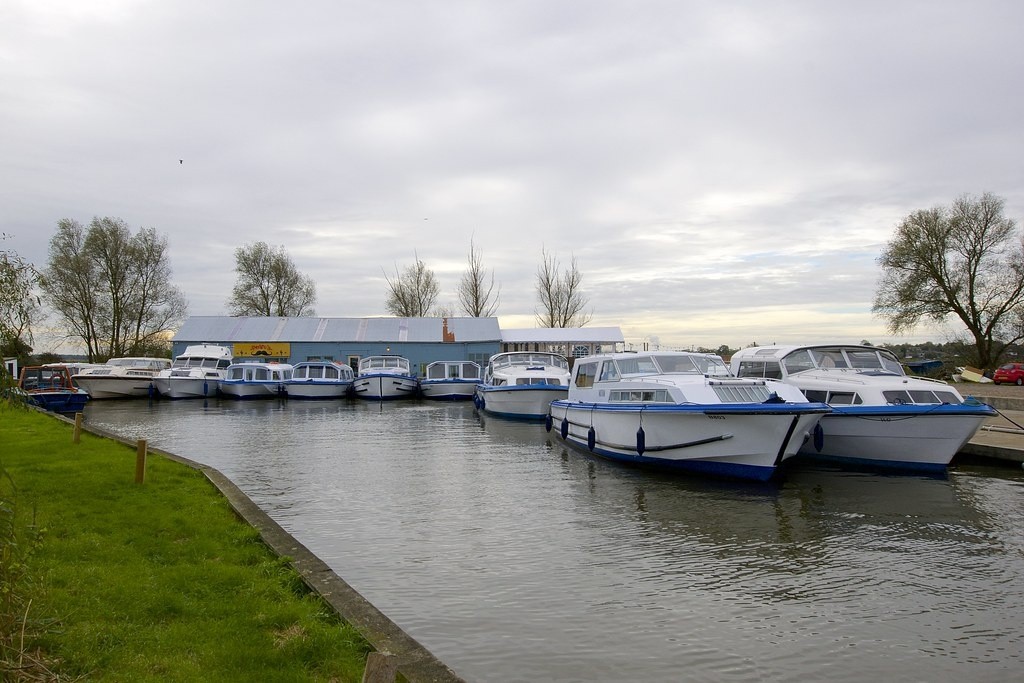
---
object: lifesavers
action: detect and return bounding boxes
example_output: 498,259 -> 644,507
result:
49,374 -> 65,387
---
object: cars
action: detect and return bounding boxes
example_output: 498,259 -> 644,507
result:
992,363 -> 1024,386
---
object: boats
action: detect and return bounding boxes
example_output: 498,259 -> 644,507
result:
69,356 -> 175,399
30,360 -> 94,386
723,342 -> 1000,473
352,355 -> 418,399
545,350 -> 833,481
215,362 -> 295,397
470,351 -> 574,420
0,365 -> 89,414
149,344 -> 233,399
280,360 -> 356,398
416,360 -> 484,399
